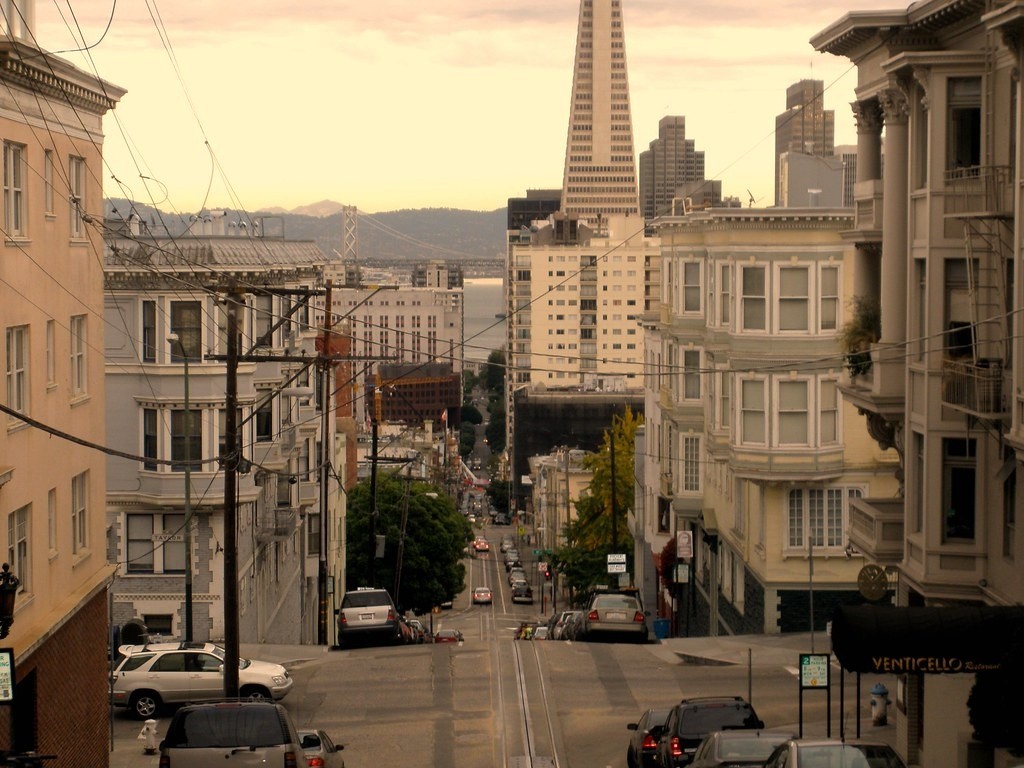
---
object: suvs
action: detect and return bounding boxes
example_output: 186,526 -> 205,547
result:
650,696 -> 765,768
109,639 -> 294,720
332,587 -> 400,651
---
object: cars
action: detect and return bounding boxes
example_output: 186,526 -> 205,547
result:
397,615 -> 464,645
295,729 -> 345,768
763,739 -> 908,768
474,534 -> 535,605
626,705 -> 672,768
472,587 -> 493,605
512,594 -> 651,640
460,495 -> 511,526
691,729 -> 799,768
157,700 -> 307,768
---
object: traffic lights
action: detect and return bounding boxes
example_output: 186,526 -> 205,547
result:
545,571 -> 551,580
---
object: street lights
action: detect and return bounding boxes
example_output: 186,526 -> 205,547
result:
165,333 -> 192,643
225,387 -> 315,702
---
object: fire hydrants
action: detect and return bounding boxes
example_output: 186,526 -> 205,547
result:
870,683 -> 892,726
